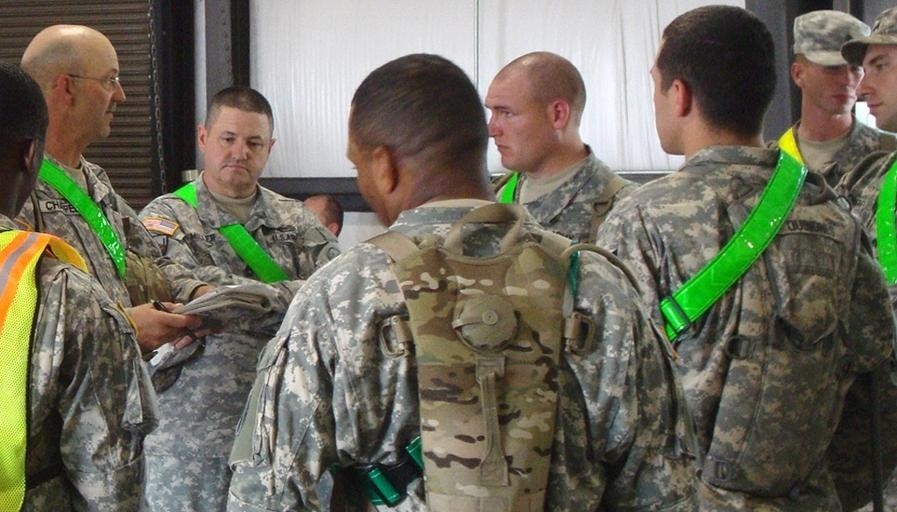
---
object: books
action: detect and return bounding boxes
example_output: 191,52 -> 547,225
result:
171,291 -> 279,321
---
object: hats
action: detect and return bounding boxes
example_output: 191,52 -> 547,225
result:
793,6 -> 897,67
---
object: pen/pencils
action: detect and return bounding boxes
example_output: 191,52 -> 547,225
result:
150,298 -> 206,348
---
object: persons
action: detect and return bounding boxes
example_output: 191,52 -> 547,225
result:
300,192 -> 343,236
226,53 -> 699,512
824,5 -> 897,510
484,51 -> 640,246
0,62 -> 160,512
19,24 -> 223,355
596,5 -> 895,511
778,8 -> 897,189
138,85 -> 345,512
